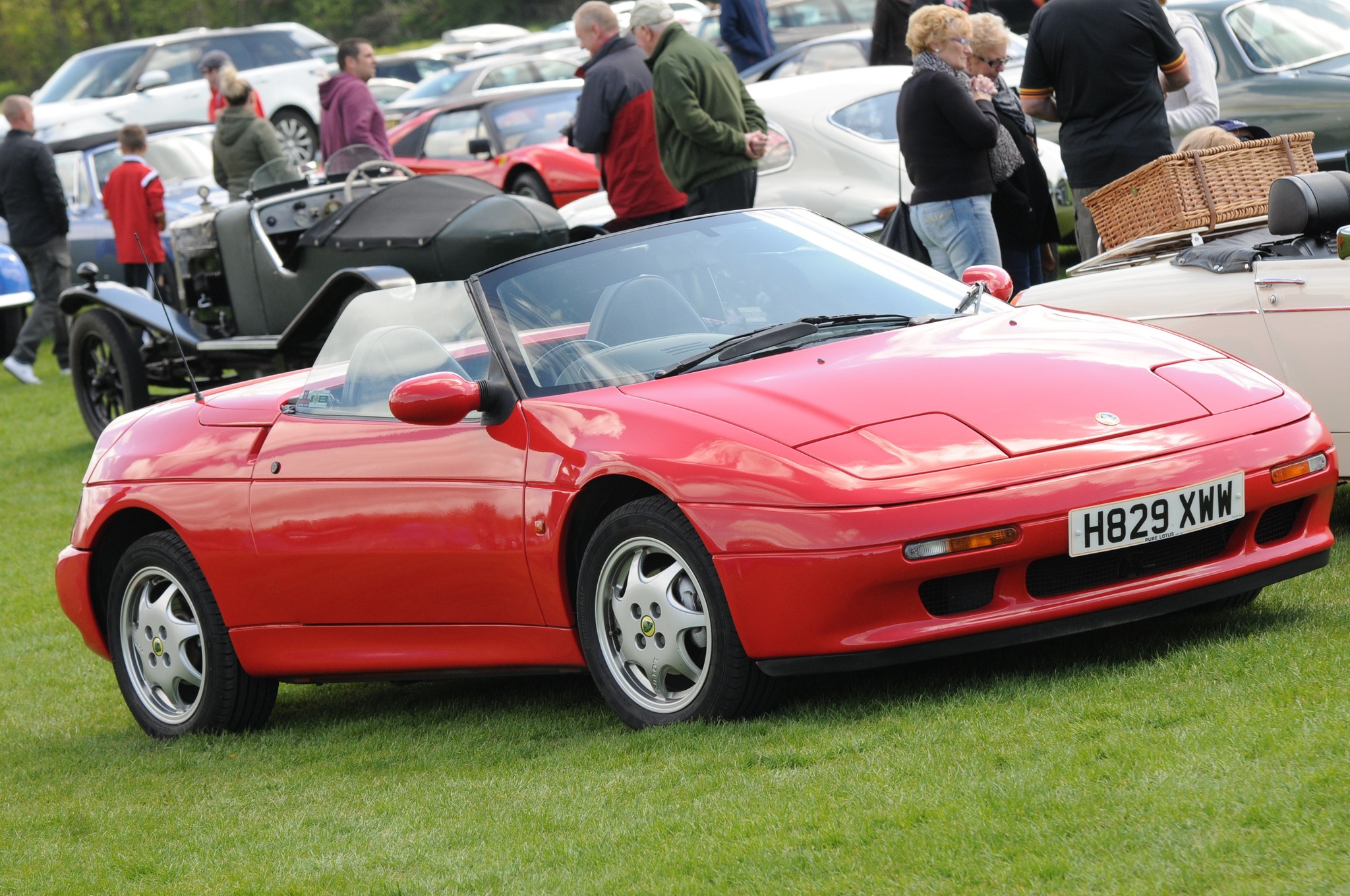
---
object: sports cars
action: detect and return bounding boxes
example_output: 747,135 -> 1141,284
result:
556,65 -> 1074,246
58,143 -> 613,443
55,206 -> 1338,739
1008,170 -> 1350,486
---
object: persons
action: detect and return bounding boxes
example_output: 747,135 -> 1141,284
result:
0,96 -> 78,383
196,48 -> 270,125
1216,120 -> 1274,141
202,75 -> 285,202
314,36 -> 392,173
565,2 -> 689,235
1152,0 -> 1222,148
895,2 -> 1006,294
718,0 -> 777,77
1021,1 -> 1194,264
957,11 -> 1062,294
99,124 -> 168,299
870,1 -> 920,65
1176,125 -> 1242,149
624,4 -> 770,216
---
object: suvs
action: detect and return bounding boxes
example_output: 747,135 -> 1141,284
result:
0,26 -> 330,166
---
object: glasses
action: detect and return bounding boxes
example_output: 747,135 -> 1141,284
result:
973,54 -> 1012,68
950,37 -> 970,46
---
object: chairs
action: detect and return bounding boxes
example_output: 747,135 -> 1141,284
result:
341,324 -> 477,420
583,272 -> 711,352
1267,171 -> 1350,258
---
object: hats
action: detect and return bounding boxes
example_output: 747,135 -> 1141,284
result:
623,2 -> 674,38
196,50 -> 233,69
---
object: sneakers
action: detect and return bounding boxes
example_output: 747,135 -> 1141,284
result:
4,356 -> 42,385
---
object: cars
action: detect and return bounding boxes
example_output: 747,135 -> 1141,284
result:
0,118 -> 228,361
1163,0 -> 1350,173
367,0 -> 878,135
380,86 -> 605,210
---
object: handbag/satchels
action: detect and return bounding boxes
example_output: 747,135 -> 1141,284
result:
876,201 -> 932,267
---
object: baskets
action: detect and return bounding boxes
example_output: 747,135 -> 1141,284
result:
1082,131 -> 1319,253
